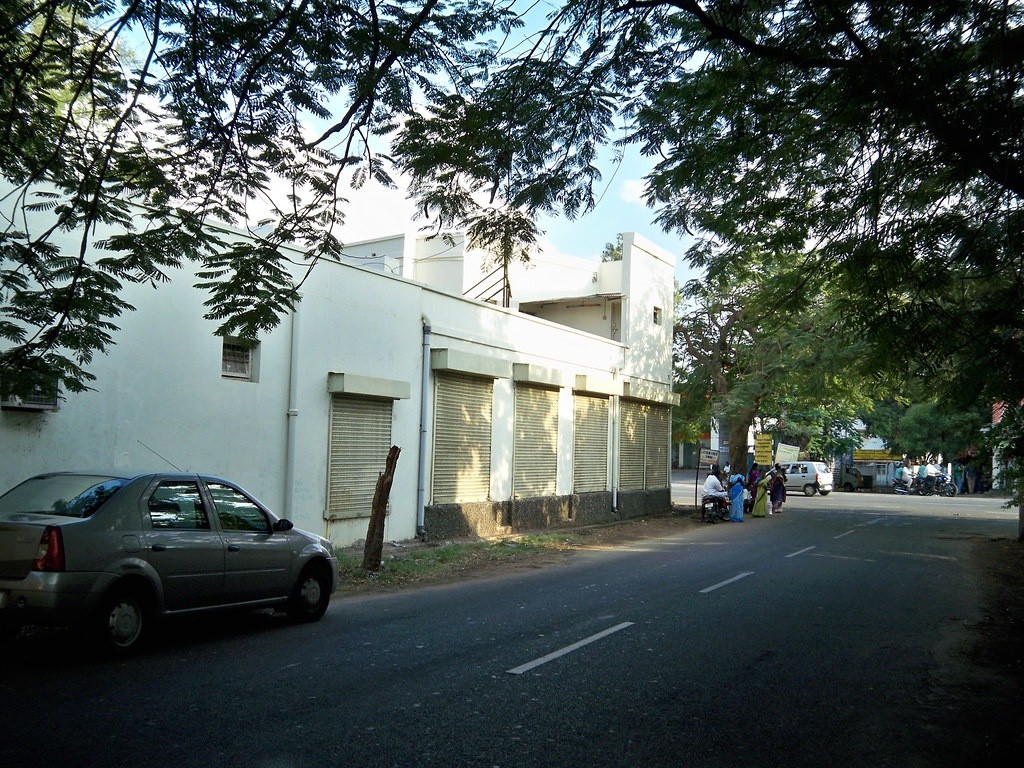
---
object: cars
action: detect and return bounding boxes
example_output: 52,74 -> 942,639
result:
765,461 -> 834,497
1,467 -> 339,655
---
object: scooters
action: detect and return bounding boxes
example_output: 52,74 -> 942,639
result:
702,485 -> 731,524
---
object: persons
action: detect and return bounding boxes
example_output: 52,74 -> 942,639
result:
707,465 -> 723,485
724,462 -> 730,479
965,459 -> 979,493
727,466 -> 746,522
744,463 -> 760,513
895,462 -> 913,492
703,470 -> 725,520
752,470 -> 770,517
771,463 -> 788,513
954,463 -> 963,494
918,460 -> 944,494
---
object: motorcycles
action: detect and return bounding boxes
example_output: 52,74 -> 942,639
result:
892,470 -> 958,497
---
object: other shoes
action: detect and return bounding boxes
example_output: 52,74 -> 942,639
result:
930,488 -> 938,492
